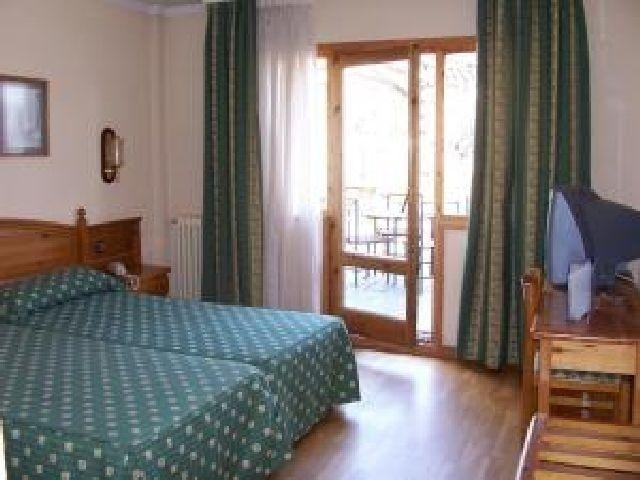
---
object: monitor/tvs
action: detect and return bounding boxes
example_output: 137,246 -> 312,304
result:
547,183 -> 640,288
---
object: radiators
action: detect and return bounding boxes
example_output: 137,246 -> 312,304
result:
169,213 -> 200,300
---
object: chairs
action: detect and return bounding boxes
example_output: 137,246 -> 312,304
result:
519,275 -> 632,438
515,333 -> 640,480
346,193 -> 459,287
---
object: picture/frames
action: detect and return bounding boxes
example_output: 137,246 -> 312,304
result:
1,75 -> 49,159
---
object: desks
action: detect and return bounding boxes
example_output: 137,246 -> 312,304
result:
535,292 -> 640,427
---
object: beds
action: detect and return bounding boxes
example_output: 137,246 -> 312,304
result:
1,206 -> 360,480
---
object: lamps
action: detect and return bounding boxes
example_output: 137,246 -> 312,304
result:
101,127 -> 125,183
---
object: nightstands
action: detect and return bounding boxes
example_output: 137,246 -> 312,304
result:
141,263 -> 172,294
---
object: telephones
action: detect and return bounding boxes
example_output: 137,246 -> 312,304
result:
106,261 -> 126,276
